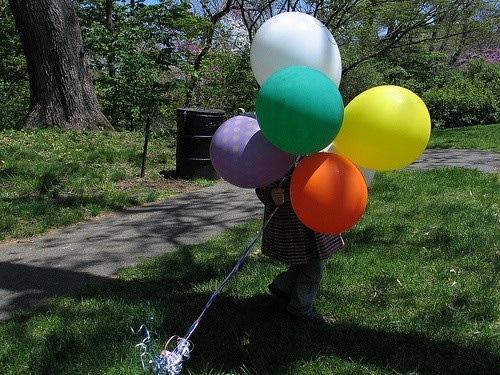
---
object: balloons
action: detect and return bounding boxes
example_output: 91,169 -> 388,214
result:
250,12 -> 342,89
256,66 -> 344,155
333,85 -> 431,172
290,152 -> 368,235
209,111 -> 296,188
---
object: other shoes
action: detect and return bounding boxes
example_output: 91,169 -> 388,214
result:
267,281 -> 325,326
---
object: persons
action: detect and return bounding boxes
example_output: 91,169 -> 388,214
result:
255,155 -> 345,325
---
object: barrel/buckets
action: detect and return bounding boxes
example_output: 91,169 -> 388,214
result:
176,107 -> 227,180
